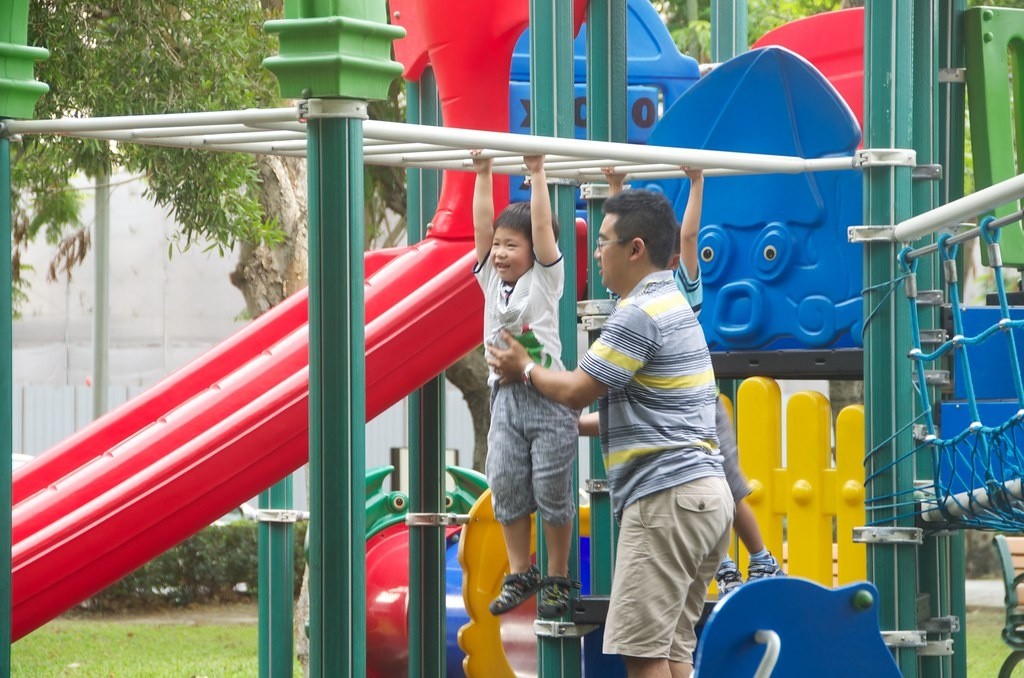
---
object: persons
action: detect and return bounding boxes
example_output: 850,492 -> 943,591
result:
486,190 -> 734,678
601,164 -> 787,599
470,148 -> 580,619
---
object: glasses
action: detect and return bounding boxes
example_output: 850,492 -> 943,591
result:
597,239 -> 624,252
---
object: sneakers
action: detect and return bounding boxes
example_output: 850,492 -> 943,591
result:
745,551 -> 785,583
715,562 -> 743,600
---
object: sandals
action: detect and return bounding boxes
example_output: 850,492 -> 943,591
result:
489,564 -> 545,615
537,569 -> 582,619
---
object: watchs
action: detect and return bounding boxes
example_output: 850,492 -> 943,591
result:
521,362 -> 535,389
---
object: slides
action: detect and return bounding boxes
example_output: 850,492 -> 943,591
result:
11,238 -> 523,643
